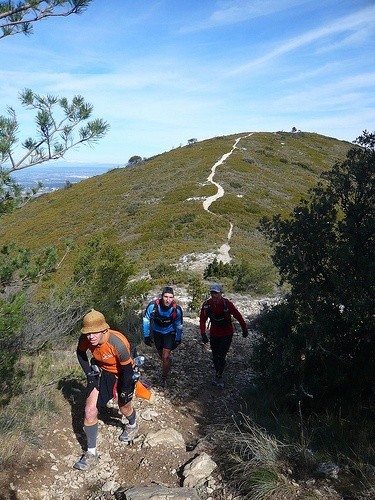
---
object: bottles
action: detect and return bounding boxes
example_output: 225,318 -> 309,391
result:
133,355 -> 145,367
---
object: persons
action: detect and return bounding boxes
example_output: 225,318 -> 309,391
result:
200,284 -> 249,389
142,287 -> 183,386
73,309 -> 140,471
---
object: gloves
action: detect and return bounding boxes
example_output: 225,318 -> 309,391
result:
175,339 -> 181,346
202,335 -> 208,344
84,376 -> 100,398
242,329 -> 249,338
144,336 -> 153,347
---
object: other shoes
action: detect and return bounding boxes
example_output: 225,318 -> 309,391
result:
73,449 -> 98,470
119,387 -> 129,404
211,374 -> 225,388
120,422 -> 139,442
161,375 -> 168,387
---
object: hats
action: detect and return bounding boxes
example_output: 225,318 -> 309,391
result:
209,284 -> 222,292
80,308 -> 110,333
